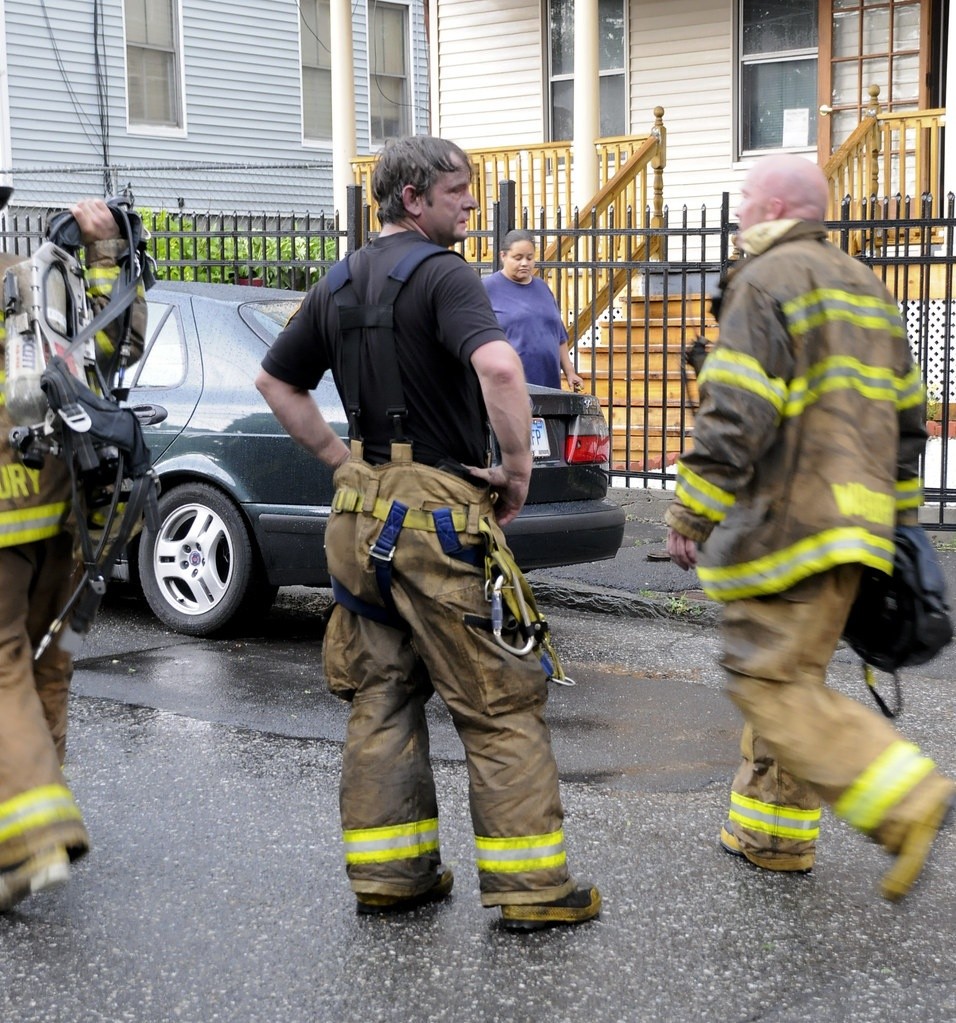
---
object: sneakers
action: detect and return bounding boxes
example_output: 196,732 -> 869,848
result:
720,822 -> 813,873
501,883 -> 602,930
357,862 -> 454,913
0,843 -> 72,912
881,778 -> 956,905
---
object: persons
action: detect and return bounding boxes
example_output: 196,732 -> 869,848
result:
482,231 -> 585,392
0,184 -> 148,915
663,155 -> 955,896
255,139 -> 604,923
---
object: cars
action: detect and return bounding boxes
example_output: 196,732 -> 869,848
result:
112,280 -> 627,637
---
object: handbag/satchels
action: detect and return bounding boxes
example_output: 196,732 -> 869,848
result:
840,523 -> 953,720
